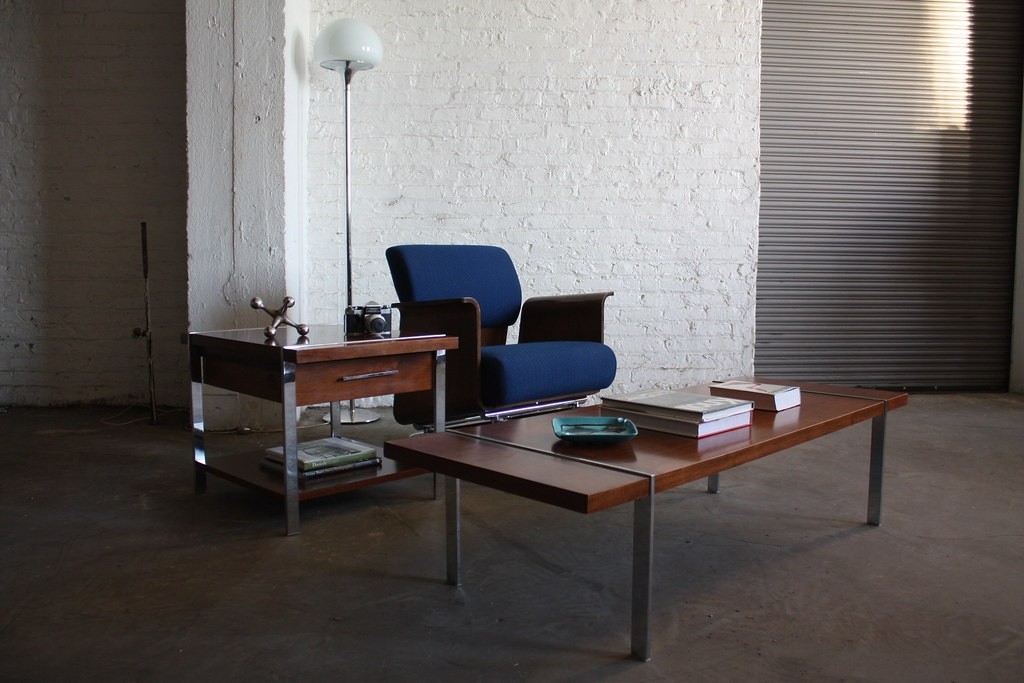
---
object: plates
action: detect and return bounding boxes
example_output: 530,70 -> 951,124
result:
551,417 -> 638,441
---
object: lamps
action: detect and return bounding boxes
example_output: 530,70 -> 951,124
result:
312,17 -> 385,425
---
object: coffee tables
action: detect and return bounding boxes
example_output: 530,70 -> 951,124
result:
383,376 -> 909,665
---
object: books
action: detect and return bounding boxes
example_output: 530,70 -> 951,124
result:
710,380 -> 801,412
257,437 -> 383,479
599,388 -> 755,440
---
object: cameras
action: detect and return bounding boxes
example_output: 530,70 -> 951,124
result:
343,300 -> 402,337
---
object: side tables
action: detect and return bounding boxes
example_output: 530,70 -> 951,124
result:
180,325 -> 458,537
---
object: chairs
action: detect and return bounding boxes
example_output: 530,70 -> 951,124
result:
386,244 -> 617,432
